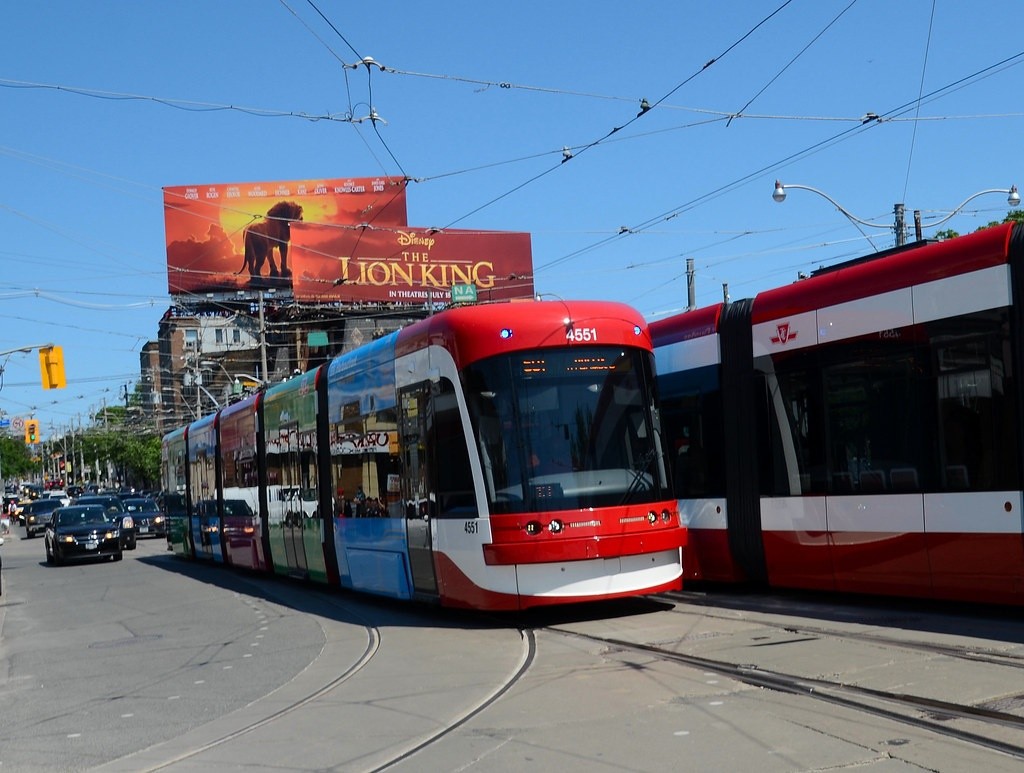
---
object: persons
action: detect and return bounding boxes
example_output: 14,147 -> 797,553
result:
354,484 -> 391,519
7,500 -> 17,525
334,487 -> 352,519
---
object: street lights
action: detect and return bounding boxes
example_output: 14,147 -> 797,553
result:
773,177 -> 1020,246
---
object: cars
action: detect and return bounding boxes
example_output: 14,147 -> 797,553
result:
43,503 -> 123,566
5,480 -> 165,550
192,498 -> 258,549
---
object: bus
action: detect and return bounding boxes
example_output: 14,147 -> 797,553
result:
158,293 -> 691,618
646,214 -> 1024,612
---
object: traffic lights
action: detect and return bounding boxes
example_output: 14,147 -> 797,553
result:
23,419 -> 40,445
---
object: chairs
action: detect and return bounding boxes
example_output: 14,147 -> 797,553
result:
835,473 -> 856,495
887,469 -> 922,494
947,465 -> 971,493
858,469 -> 888,495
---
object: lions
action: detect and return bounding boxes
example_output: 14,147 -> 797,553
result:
232,200 -> 305,280
